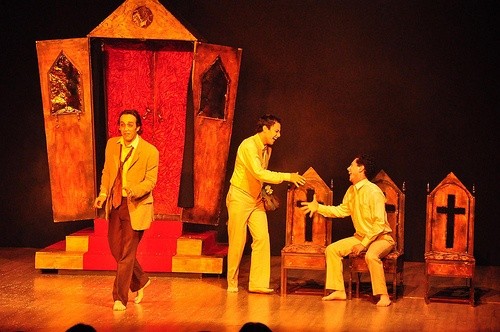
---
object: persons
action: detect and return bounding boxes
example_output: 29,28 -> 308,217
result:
239,322 -> 272,332
94,109 -> 160,311
226,113 -> 306,293
66,323 -> 96,332
300,155 -> 395,306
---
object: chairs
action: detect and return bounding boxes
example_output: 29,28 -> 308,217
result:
424,172 -> 476,307
281,167 -> 334,295
348,169 -> 406,301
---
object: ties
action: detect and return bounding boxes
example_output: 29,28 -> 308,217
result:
112,144 -> 134,209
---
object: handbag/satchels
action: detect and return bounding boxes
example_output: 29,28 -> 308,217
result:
264,195 -> 281,213
